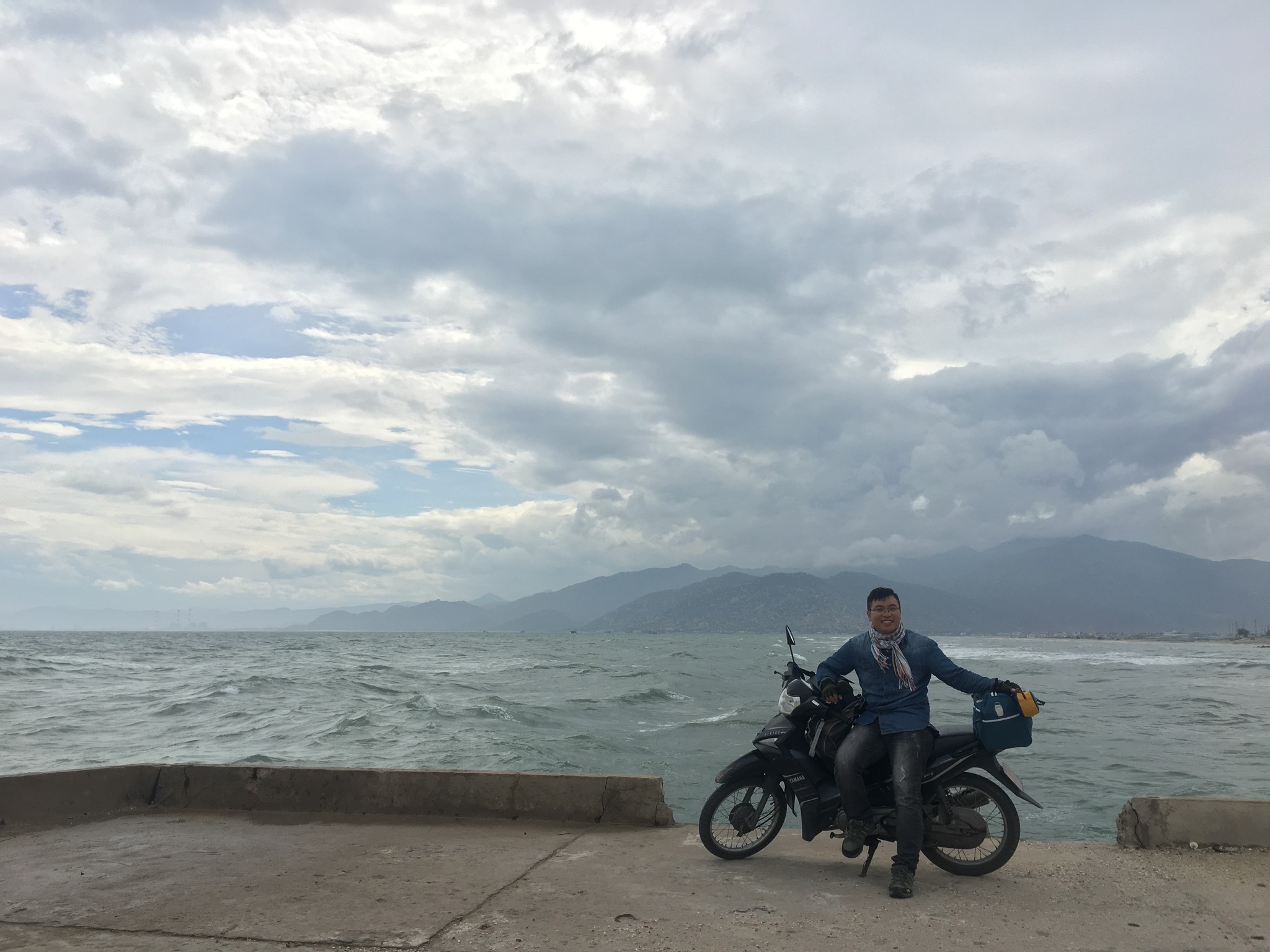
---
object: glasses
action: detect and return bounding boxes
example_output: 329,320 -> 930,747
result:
869,605 -> 900,614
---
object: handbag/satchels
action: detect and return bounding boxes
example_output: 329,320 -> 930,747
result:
1017,688 -> 1046,717
972,692 -> 1033,756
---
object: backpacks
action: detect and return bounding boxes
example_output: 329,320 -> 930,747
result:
805,694 -> 864,770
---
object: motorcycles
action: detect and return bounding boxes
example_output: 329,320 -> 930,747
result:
698,624 -> 1046,877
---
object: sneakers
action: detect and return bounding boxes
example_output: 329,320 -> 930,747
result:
888,865 -> 916,898
842,819 -> 875,858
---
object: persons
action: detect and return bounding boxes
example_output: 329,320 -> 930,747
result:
815,585 -> 1022,899
